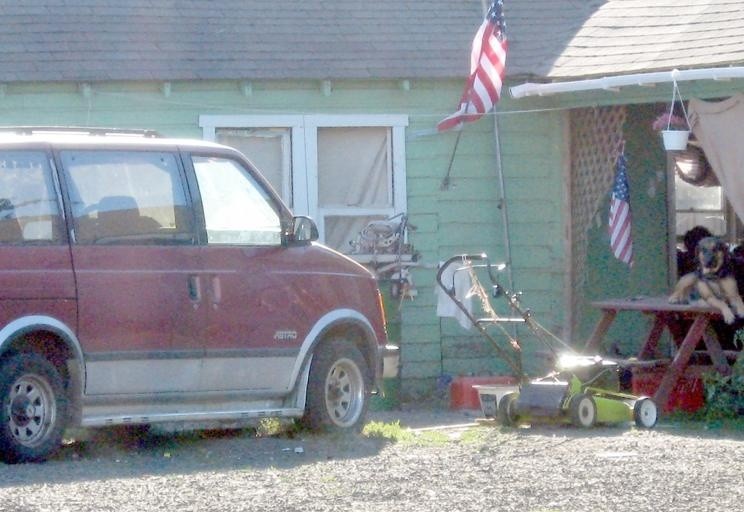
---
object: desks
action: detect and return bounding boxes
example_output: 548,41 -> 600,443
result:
581,295 -> 736,413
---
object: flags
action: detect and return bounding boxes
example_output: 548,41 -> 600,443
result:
436,0 -> 509,133
608,158 -> 635,270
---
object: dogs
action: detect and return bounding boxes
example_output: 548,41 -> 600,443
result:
669,236 -> 744,325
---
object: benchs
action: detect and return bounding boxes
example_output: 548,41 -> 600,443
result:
692,347 -> 740,359
530,347 -> 672,377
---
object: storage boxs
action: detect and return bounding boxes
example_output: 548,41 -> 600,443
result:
449,375 -> 519,411
631,369 -> 708,414
472,382 -> 521,419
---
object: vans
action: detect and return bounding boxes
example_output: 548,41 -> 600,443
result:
0,125 -> 403,462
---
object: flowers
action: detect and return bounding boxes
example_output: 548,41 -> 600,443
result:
650,110 -> 691,131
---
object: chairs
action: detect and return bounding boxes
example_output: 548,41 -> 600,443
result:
1,196 -> 24,244
91,193 -> 162,242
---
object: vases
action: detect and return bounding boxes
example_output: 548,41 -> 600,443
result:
661,129 -> 691,151
